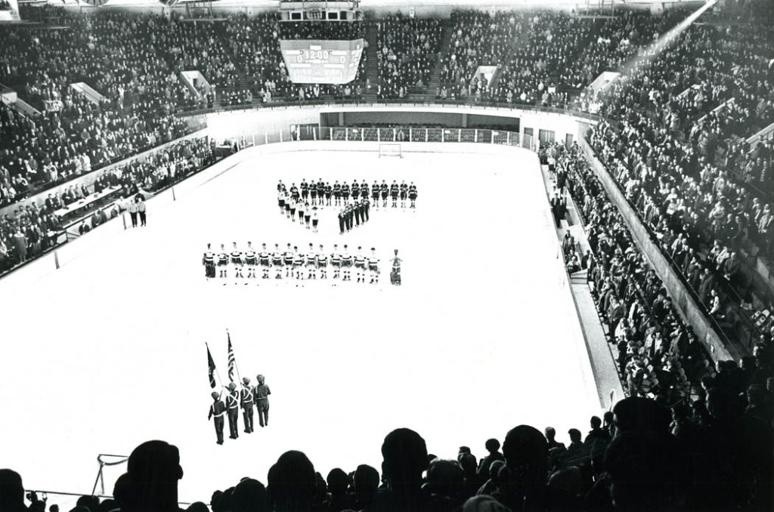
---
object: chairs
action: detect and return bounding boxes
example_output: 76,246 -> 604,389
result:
0,7 -> 242,282
544,2 -> 774,418
241,11 -> 543,111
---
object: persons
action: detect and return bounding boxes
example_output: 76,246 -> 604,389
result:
0,0 -> 774,510
